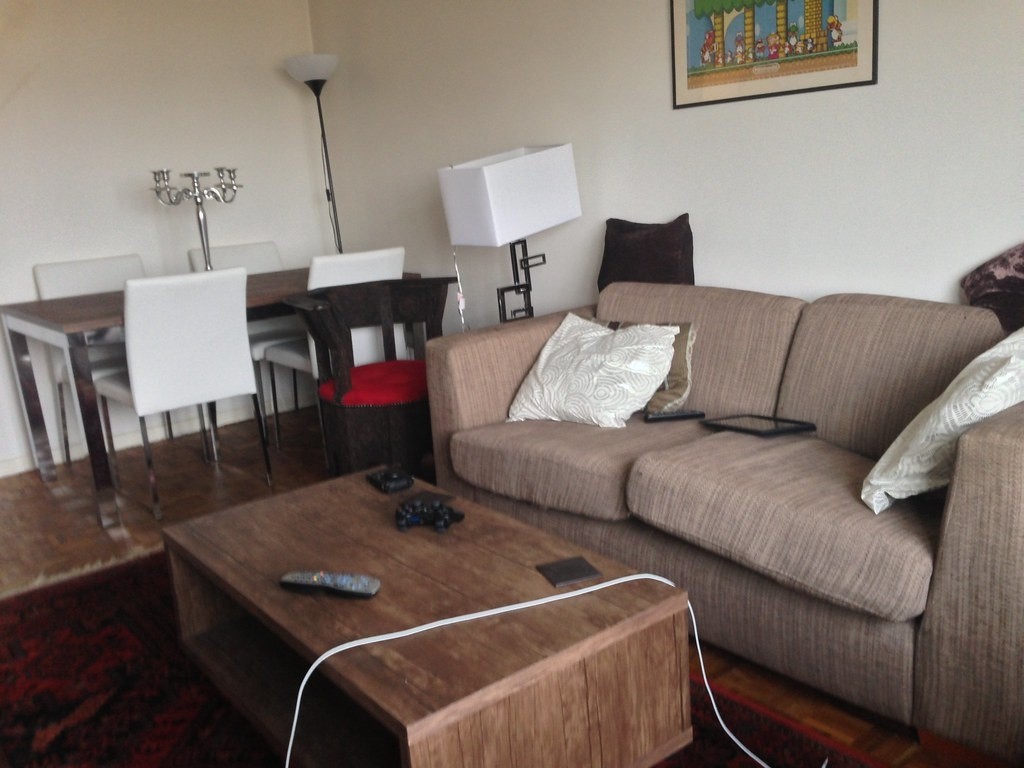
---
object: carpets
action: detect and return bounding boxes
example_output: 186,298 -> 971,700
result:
0,539 -> 889,768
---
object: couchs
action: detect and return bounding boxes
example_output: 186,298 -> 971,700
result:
424,281 -> 1024,768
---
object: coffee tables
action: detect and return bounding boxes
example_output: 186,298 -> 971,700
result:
159,462 -> 695,768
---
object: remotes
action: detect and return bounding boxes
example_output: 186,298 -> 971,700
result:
281,572 -> 380,598
644,410 -> 705,423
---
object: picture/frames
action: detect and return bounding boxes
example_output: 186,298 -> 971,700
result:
670,0 -> 879,109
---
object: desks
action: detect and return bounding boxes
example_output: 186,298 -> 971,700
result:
0,267 -> 423,529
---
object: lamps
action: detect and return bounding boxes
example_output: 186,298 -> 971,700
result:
283,53 -> 344,254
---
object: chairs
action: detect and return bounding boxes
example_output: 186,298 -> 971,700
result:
264,246 -> 409,472
279,275 -> 459,476
187,241 -> 307,443
32,253 -> 209,487
93,265 -> 275,521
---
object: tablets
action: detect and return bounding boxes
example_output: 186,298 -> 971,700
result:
702,414 -> 817,438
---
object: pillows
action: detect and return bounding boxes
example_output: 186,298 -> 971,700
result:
585,318 -> 696,415
505,312 -> 680,429
860,326 -> 1024,516
960,241 -> 1024,338
597,212 -> 695,293
